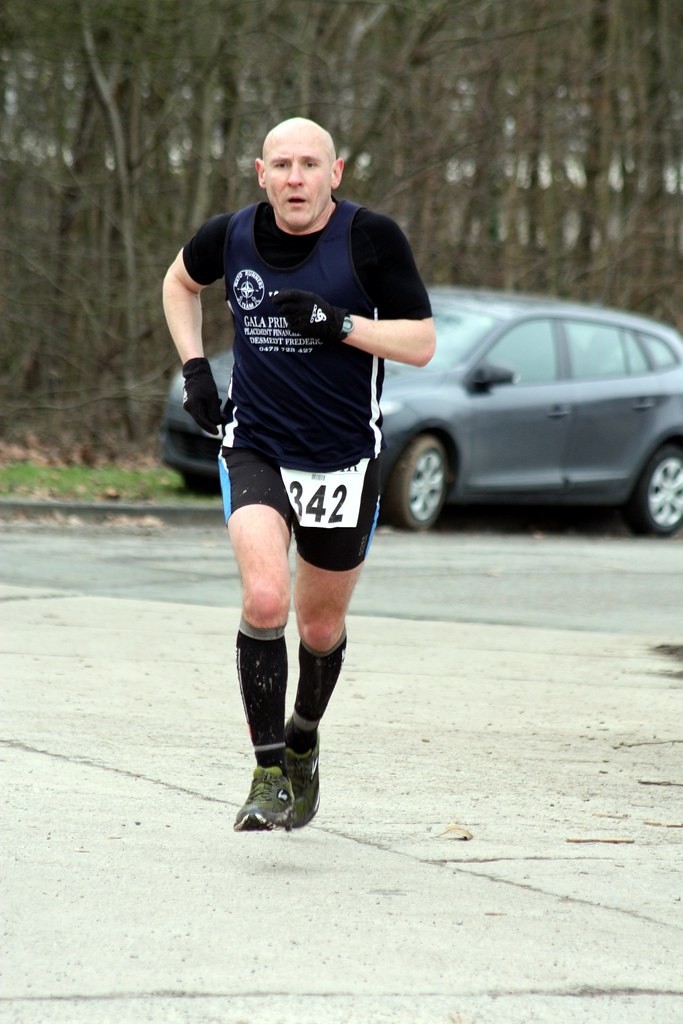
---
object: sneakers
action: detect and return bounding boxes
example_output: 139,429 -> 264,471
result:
233,765 -> 295,832
285,715 -> 320,828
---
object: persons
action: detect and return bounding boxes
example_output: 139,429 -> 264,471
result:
161,117 -> 437,831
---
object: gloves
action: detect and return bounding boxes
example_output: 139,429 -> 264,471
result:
272,288 -> 348,343
182,358 -> 222,435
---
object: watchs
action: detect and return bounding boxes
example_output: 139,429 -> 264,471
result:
339,309 -> 354,342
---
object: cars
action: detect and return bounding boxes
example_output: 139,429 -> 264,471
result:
159,288 -> 683,539
